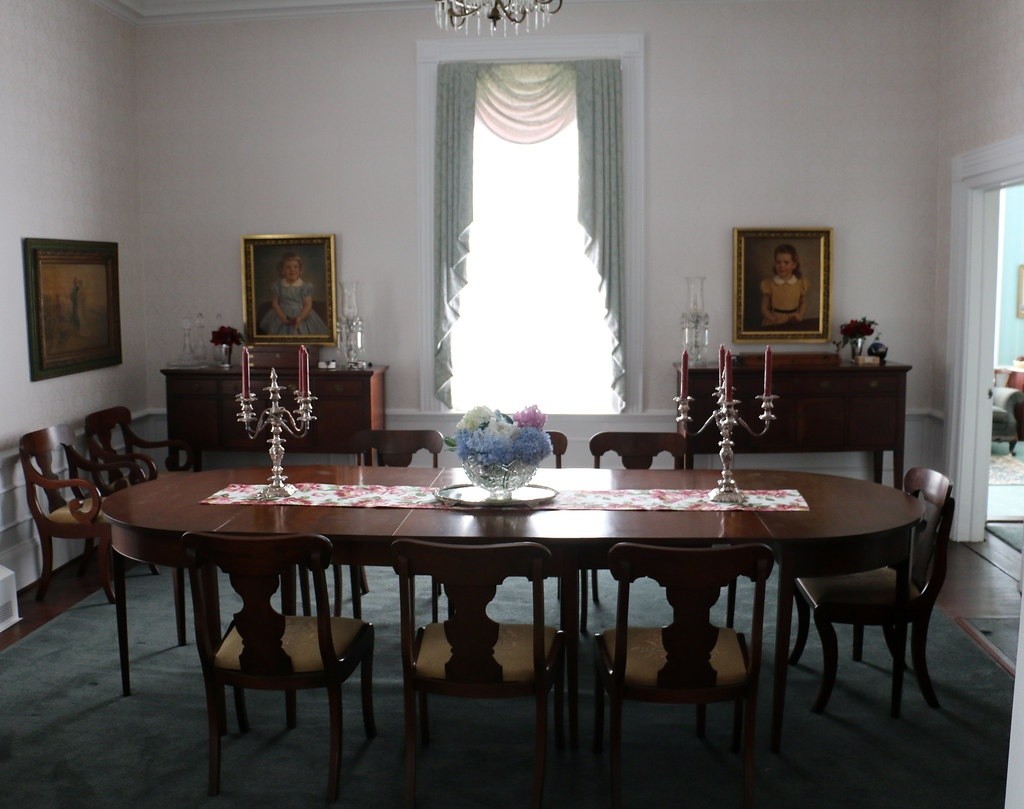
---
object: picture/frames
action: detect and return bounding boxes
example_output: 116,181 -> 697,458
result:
24,238 -> 122,382
239,233 -> 337,348
731,226 -> 832,345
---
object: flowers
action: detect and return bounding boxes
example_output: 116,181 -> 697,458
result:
210,325 -> 244,346
838,316 -> 879,348
443,405 -> 555,467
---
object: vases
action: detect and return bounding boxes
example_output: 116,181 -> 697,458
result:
463,463 -> 538,496
851,338 -> 863,359
221,344 -> 231,364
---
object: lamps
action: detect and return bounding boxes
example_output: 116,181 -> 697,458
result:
434,0 -> 562,39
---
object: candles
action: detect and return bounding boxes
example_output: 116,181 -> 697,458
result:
242,346 -> 251,398
298,344 -> 309,397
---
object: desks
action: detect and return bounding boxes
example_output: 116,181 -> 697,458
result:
101,468 -> 927,757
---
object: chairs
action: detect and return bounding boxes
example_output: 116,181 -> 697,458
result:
182,429 -> 956,808
992,388 -> 1024,456
19,406 -> 195,605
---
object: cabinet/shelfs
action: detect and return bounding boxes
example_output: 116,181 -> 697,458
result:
675,361 -> 912,491
161,361 -> 387,468
